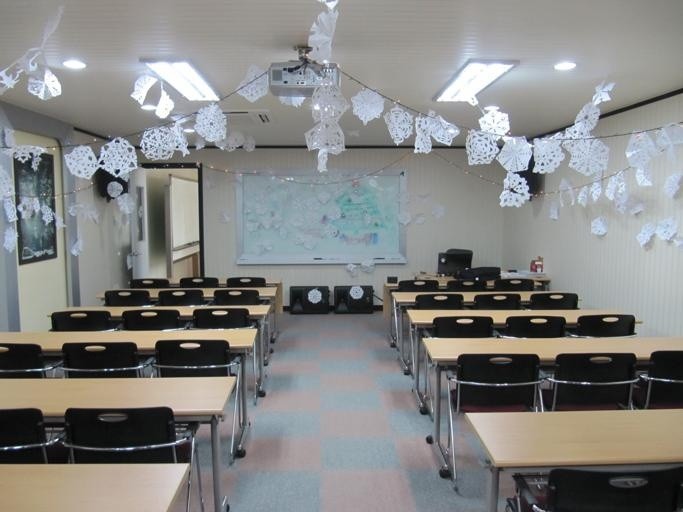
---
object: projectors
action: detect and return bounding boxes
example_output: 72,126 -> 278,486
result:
267,60 -> 341,96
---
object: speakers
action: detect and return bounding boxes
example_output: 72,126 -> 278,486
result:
333,286 -> 373,313
96,156 -> 128,201
290,285 -> 329,314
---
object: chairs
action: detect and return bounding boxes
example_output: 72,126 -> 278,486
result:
417,316 -> 492,423
494,279 -> 534,291
190,309 -> 258,405
473,294 -> 521,310
105,290 -> 150,306
121,310 -> 191,331
0,342 -> 63,378
495,316 -> 567,339
505,465 -> 682,512
180,277 -> 219,287
407,294 -> 464,379
49,310 -> 122,332
130,278 -> 169,287
524,293 -> 578,309
214,290 -> 260,305
391,280 -> 439,351
568,314 -> 637,338
437,352 -> 545,492
637,351 -> 682,409
61,407 -> 206,512
446,279 -> 487,291
0,409 -> 71,464
539,353 -> 640,410
150,340 -> 242,464
158,290 -> 204,306
227,277 -> 266,287
61,341 -> 155,379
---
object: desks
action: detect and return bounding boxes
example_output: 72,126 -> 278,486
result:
464,408 -> 683,512
383,275 -> 551,348
0,463 -> 190,512
421,337 -> 682,478
46,304 -> 272,398
391,291 -> 583,375
96,287 -> 278,366
0,328 -> 258,459
407,310 -> 642,414
127,278 -> 283,342
1,376 -> 237,512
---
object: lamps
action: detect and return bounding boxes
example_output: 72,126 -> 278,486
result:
432,58 -> 519,103
140,57 -> 219,102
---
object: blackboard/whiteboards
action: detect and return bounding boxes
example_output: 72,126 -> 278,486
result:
170,174 -> 200,258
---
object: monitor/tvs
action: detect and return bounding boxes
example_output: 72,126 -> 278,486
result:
438,251 -> 472,278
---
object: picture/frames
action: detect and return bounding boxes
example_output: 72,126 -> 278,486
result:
14,152 -> 58,266
